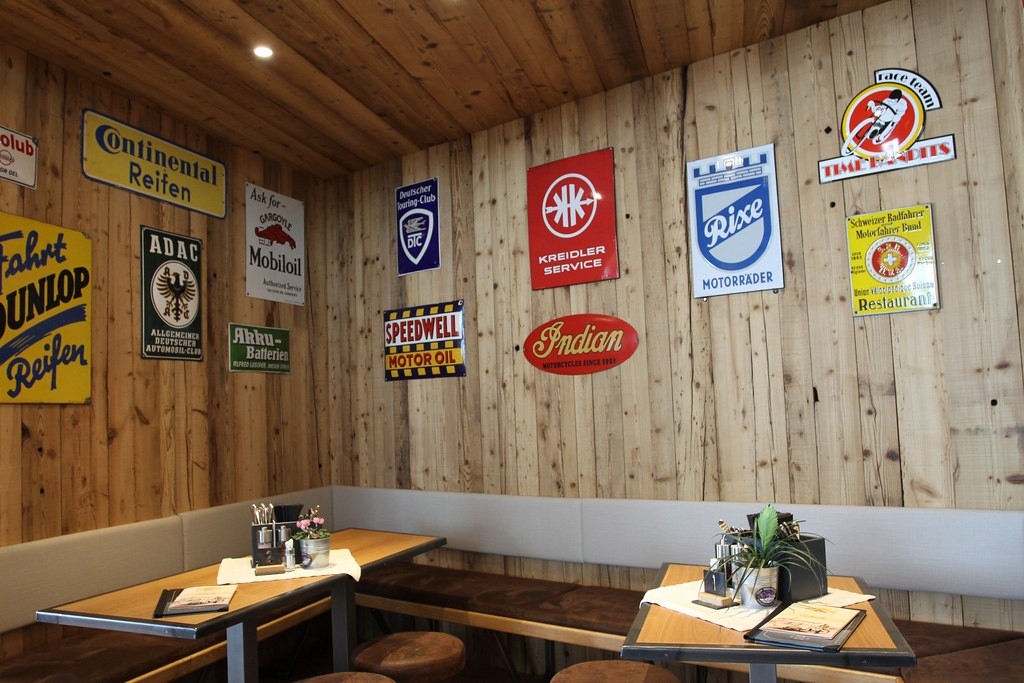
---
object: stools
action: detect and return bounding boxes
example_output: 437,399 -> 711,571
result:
302,671 -> 391,682
549,659 -> 682,683
354,631 -> 466,683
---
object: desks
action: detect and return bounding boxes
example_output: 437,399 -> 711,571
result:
620,561 -> 918,682
35,526 -> 448,682
704,508 -> 833,607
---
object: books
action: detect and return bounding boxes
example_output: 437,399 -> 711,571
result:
743,600 -> 867,653
152,583 -> 238,618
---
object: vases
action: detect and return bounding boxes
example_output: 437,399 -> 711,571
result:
300,536 -> 332,569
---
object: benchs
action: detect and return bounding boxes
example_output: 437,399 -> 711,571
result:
0,484 -> 1024,682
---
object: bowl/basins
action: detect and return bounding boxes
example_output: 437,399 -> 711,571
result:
273,504 -> 304,522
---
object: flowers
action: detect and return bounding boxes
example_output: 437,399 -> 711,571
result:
293,516 -> 331,540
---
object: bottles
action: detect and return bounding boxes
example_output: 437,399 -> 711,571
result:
730,538 -> 749,587
277,525 -> 292,547
285,548 -> 295,569
714,534 -> 731,586
257,526 -> 272,549
